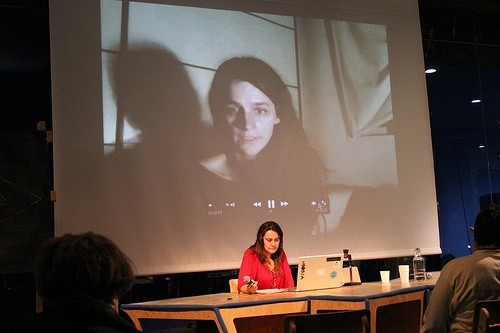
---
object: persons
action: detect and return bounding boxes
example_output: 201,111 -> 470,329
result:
419,205 -> 500,333
8,231 -> 143,333
237,221 -> 294,293
170,56 -> 331,241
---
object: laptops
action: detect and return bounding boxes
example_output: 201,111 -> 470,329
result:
283,254 -> 344,292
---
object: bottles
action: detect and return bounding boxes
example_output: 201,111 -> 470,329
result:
413,246 -> 426,283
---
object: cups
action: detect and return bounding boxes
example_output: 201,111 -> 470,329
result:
399,265 -> 409,283
380,270 -> 390,283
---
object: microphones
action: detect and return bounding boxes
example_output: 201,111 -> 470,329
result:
243,276 -> 255,287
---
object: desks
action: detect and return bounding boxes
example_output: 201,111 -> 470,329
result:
122,272 -> 441,333
